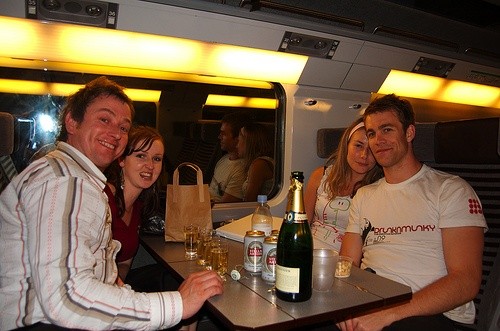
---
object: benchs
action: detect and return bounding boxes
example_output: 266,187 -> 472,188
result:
413,117 -> 500,331
0,112 -> 18,196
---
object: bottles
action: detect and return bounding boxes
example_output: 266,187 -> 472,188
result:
276,171 -> 312,302
251,196 -> 273,236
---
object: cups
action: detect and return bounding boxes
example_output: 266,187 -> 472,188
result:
313,248 -> 339,292
198,229 -> 211,264
183,224 -> 198,254
206,232 -> 218,271
336,256 -> 353,277
210,238 -> 227,281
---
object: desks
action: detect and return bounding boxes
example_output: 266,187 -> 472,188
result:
139,231 -> 412,331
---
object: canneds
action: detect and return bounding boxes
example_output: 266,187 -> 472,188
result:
244,231 -> 266,273
262,236 -> 277,282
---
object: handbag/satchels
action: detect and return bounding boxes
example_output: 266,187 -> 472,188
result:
164,162 -> 213,242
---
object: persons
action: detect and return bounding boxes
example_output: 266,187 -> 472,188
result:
336,93 -> 489,331
209,118 -> 275,203
0,76 -> 223,331
303,117 -> 384,253
